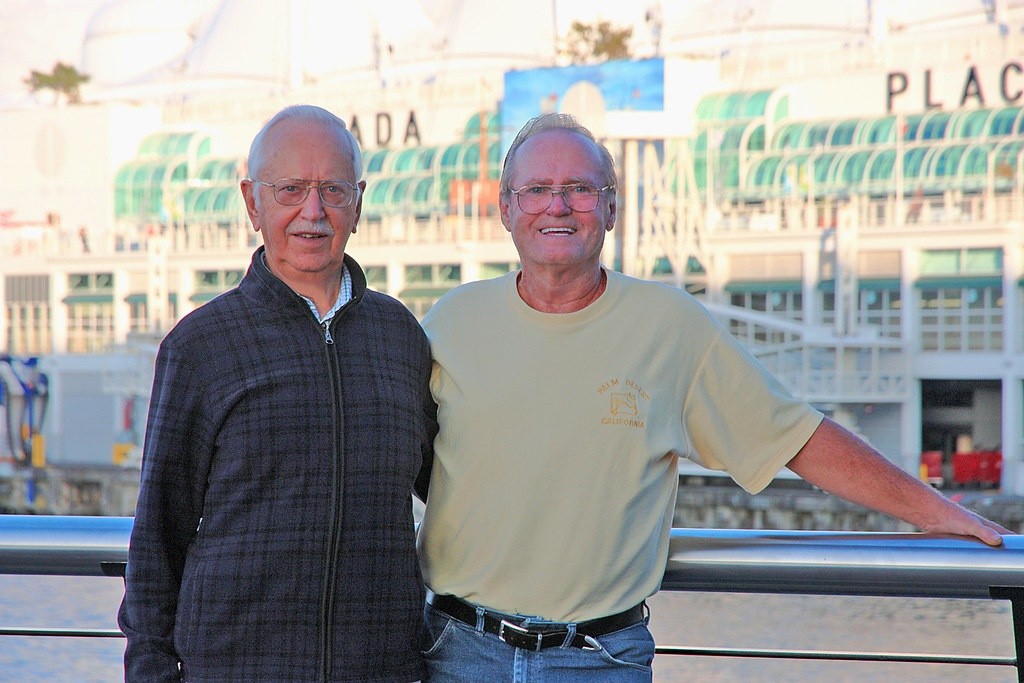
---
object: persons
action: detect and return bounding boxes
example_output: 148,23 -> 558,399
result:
418,113 -> 1013,683
117,105 -> 438,683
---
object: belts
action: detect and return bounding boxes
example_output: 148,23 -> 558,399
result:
426,588 -> 646,652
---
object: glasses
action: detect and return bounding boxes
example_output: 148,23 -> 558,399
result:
504,184 -> 610,214
251,177 -> 359,207
